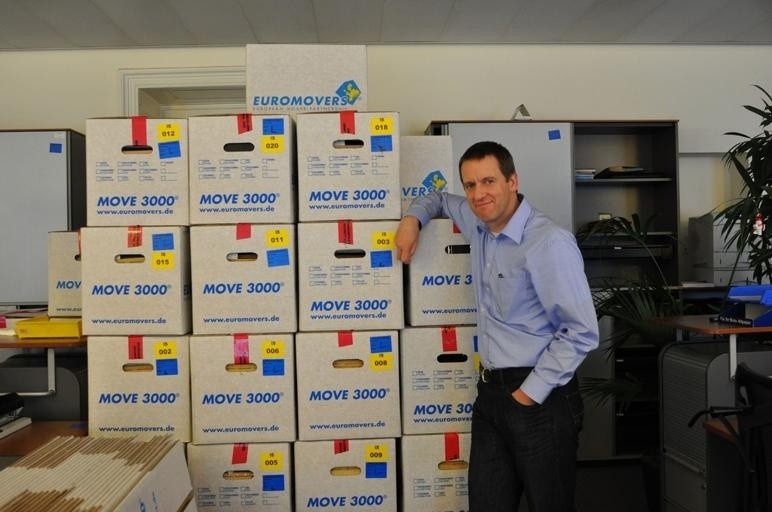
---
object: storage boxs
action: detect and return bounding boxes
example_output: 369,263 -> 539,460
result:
86,335 -> 191,443
186,224 -> 299,336
293,438 -> 397,512
190,334 -> 296,446
293,329 -> 403,442
48,230 -> 83,317
246,45 -> 368,115
296,111 -> 402,224
186,443 -> 292,512
400,433 -> 473,511
79,226 -> 191,335
187,114 -> 295,224
296,220 -> 405,332
407,218 -> 480,327
400,135 -> 453,217
399,326 -> 479,435
85,116 -> 190,228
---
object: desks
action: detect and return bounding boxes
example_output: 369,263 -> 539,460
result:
2,334 -> 86,429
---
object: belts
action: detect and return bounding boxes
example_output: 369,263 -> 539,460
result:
479,367 -> 538,386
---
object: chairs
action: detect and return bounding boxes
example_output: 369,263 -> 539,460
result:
688,363 -> 770,511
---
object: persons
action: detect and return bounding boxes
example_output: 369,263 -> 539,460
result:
394,139 -> 600,511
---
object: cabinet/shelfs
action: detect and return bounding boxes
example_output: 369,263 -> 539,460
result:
1,125 -> 84,311
686,215 -> 769,284
570,119 -> 682,285
655,311 -> 771,512
424,111 -> 576,246
573,287 -> 745,462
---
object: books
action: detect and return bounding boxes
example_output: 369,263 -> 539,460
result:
573,165 -> 645,180
